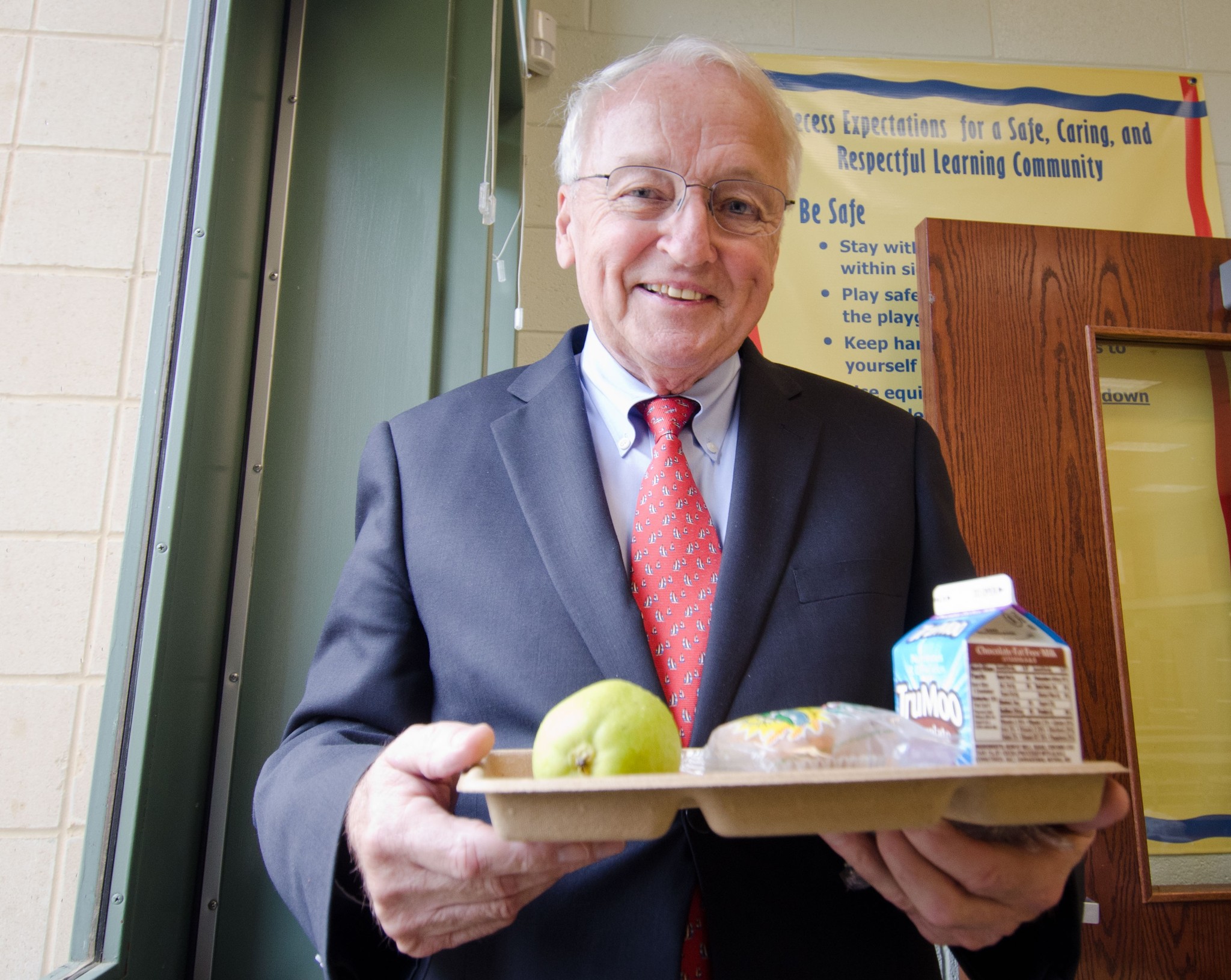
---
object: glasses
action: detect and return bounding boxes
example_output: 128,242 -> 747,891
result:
564,164 -> 797,236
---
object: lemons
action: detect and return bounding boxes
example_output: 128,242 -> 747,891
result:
530,678 -> 683,779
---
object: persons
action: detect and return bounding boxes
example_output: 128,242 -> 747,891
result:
252,36 -> 1131,980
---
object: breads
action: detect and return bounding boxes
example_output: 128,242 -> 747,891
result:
702,704 -> 907,776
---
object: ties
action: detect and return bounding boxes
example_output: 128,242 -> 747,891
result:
629,398 -> 723,979
